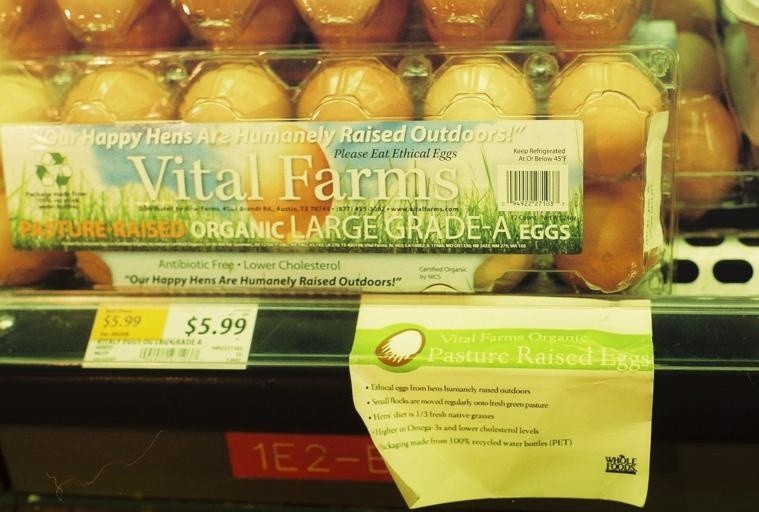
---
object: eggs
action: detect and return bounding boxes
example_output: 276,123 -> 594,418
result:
0,0 -> 759,293
